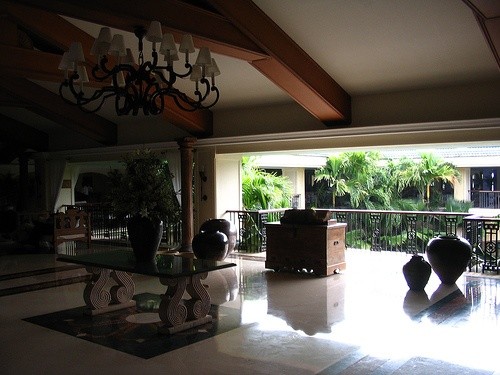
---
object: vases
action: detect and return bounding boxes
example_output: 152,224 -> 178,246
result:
403,255 -> 431,290
426,236 -> 472,284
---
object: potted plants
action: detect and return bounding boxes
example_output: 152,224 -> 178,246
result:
104,145 -> 183,261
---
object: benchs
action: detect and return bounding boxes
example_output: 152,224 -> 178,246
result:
17,211 -> 91,252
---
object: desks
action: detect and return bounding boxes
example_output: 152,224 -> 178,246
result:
264,209 -> 348,276
56,251 -> 237,336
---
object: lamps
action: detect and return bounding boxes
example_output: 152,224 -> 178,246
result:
58,20 -> 221,118
